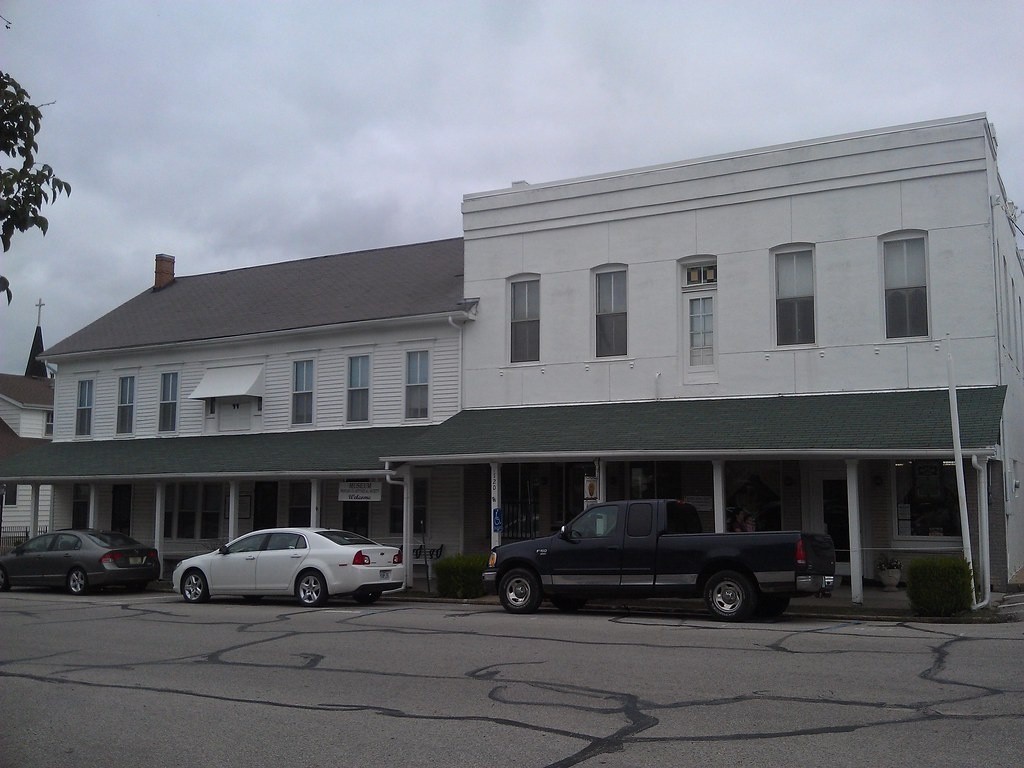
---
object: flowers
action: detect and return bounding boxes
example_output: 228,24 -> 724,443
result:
875,557 -> 904,569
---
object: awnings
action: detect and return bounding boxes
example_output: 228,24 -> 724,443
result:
0,422 -> 439,485
379,386 -> 1010,463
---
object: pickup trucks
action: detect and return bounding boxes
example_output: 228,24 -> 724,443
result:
481,498 -> 837,622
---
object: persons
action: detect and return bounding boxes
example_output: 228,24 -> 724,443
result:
728,507 -> 755,533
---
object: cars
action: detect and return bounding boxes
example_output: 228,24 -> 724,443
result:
171,526 -> 407,607
0,527 -> 161,596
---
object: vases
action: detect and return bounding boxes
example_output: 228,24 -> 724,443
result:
874,570 -> 901,591
